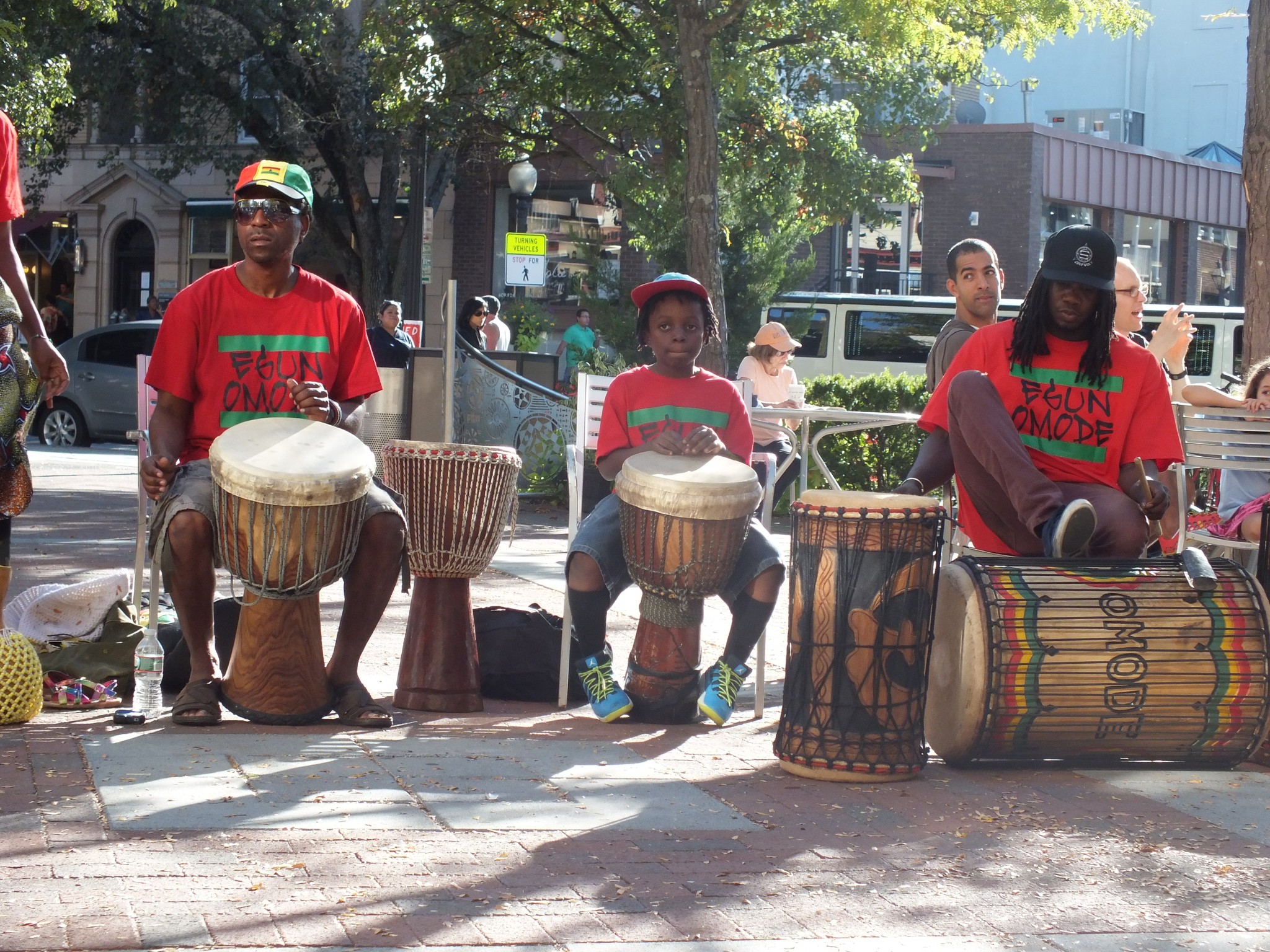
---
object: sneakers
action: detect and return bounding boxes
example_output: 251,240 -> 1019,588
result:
697,655 -> 753,726
1042,499 -> 1097,558
575,651 -> 633,723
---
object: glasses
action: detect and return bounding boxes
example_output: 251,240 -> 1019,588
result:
775,349 -> 791,356
232,198 -> 302,225
474,311 -> 488,317
579,315 -> 590,319
1115,284 -> 1148,297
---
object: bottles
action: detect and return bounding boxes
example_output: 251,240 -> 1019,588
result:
132,629 -> 165,718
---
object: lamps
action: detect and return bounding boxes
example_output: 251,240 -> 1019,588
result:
71,236 -> 85,274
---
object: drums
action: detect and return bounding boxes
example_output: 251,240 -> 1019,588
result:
614,450 -> 763,723
380,439 -> 523,712
925,556 -> 1270,770
772,489 -> 946,782
207,417 -> 377,721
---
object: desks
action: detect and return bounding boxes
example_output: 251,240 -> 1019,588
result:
745,403 -> 922,498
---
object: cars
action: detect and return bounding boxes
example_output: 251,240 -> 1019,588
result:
19,319 -> 164,448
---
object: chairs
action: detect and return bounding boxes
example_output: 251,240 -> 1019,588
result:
555,367 -> 781,723
131,347 -> 163,639
1165,397 -> 1270,559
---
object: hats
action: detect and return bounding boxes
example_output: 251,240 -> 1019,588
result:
1041,223 -> 1117,290
755,322 -> 802,352
631,272 -> 714,318
234,160 -> 313,214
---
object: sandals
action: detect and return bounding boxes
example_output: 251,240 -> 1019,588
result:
42,671 -> 73,701
47,679 -> 122,709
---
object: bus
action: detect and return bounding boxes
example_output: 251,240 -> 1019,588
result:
759,289 -> 1245,419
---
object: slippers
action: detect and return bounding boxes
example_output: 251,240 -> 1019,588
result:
332,685 -> 393,727
172,679 -> 222,724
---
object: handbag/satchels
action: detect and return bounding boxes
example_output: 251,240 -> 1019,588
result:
470,603 -> 613,704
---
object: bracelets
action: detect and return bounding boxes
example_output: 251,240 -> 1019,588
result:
26,335 -> 53,350
901,477 -> 924,495
1169,366 -> 1188,380
328,397 -> 342,427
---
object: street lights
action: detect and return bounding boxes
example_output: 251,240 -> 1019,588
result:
506,152 -> 537,341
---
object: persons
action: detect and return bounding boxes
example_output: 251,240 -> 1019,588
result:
367,295 -> 602,386
891,224 -> 1270,561
140,160 -> 411,730
563,273 -> 784,725
736,322 -> 802,521
0,110 -> 171,618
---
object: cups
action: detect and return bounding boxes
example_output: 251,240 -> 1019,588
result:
789,384 -> 805,407
